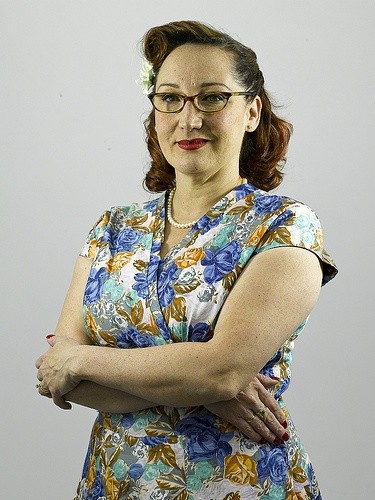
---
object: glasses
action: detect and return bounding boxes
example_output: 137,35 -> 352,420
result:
148,88 -> 254,113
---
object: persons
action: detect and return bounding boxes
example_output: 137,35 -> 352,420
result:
34,20 -> 338,500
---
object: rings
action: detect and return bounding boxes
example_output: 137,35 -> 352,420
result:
256,407 -> 267,417
248,416 -> 254,423
38,384 -> 48,393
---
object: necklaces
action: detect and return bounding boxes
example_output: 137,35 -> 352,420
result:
166,178 -> 247,228
